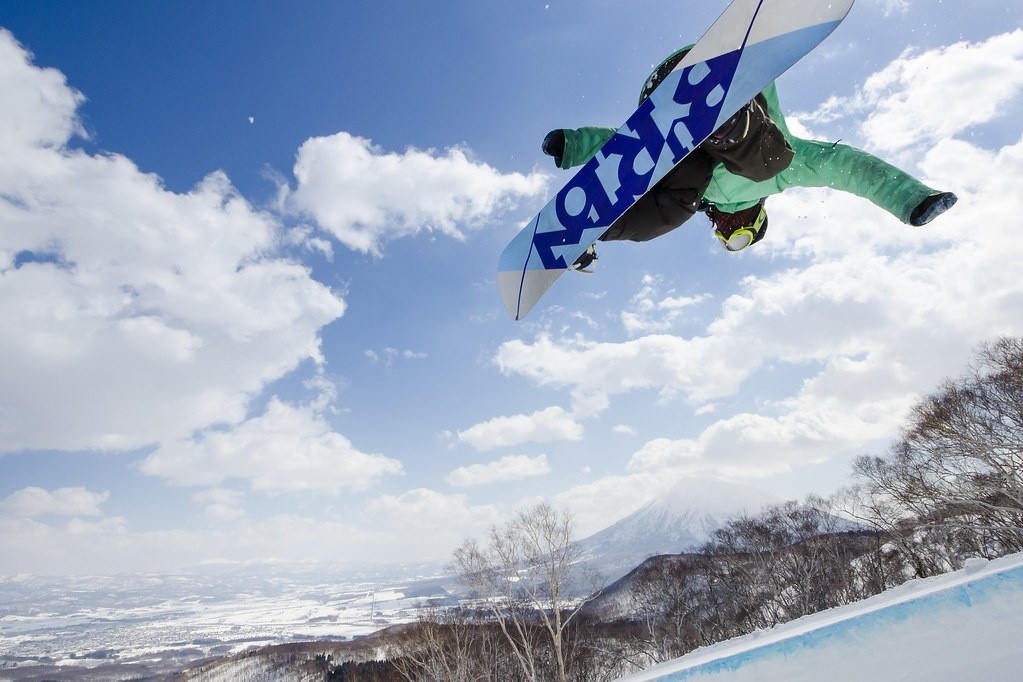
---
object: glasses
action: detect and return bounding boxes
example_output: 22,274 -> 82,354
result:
715,227 -> 755,253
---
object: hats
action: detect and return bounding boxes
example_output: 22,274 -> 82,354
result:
718,204 -> 767,245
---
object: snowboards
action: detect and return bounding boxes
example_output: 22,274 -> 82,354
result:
495,1 -> 854,324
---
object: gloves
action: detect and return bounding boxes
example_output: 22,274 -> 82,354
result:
911,193 -> 956,225
541,128 -> 565,167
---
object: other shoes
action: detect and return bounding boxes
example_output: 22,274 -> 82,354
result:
572,244 -> 598,271
705,107 -> 752,149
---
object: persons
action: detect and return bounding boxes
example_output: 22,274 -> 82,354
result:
542,42 -> 958,273
